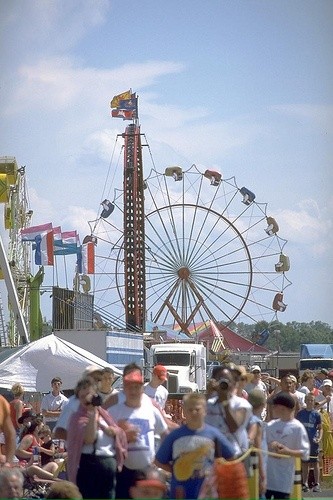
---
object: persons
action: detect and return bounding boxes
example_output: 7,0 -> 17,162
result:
0,364 -> 332,500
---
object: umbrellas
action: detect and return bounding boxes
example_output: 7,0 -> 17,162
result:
1,333 -> 126,393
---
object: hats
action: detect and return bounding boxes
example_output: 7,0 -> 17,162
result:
125,369 -> 143,384
18,411 -> 38,424
82,365 -> 105,377
152,365 -> 168,381
321,368 -> 329,375
289,375 -> 297,383
136,469 -> 167,494
323,379 -> 332,387
250,365 -> 261,374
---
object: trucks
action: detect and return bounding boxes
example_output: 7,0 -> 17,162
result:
140,343 -> 209,398
53,329 -> 145,390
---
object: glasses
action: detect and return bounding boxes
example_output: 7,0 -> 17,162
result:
40,434 -> 48,440
253,371 -> 260,374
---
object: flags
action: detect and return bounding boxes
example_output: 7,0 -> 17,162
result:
20,223 -> 97,276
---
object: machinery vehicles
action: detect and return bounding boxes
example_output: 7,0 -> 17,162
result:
296,343 -> 333,379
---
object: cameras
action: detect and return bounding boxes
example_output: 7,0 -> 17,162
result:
92,394 -> 102,407
217,378 -> 230,390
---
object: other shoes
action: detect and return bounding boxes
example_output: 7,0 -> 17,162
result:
302,484 -> 309,492
313,485 -> 320,492
